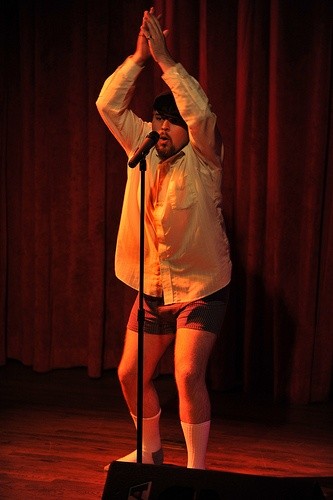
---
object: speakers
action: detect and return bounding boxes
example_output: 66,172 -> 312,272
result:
100,461 -> 333,500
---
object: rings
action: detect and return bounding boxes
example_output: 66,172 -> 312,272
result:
146,35 -> 152,40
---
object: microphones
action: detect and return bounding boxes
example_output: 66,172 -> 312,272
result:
129,131 -> 159,168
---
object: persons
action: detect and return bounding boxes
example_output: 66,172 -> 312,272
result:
94,4 -> 235,472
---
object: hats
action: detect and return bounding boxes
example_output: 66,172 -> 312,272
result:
153,89 -> 185,122
